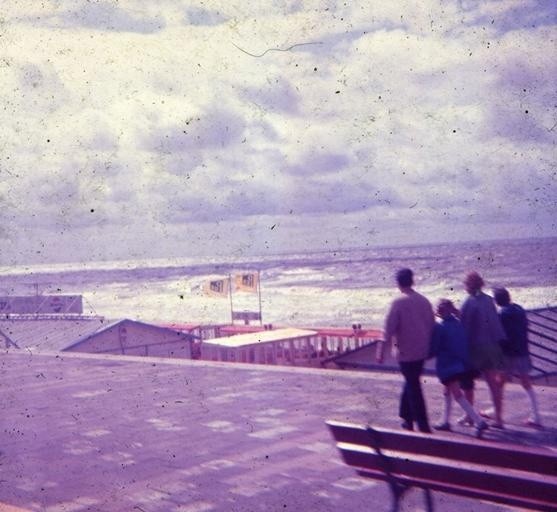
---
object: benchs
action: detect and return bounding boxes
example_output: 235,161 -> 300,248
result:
324,418 -> 557,510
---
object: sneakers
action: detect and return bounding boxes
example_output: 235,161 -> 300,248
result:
456,409 -> 504,429
433,422 -> 451,430
475,421 -> 487,439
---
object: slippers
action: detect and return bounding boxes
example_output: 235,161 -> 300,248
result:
522,418 -> 543,428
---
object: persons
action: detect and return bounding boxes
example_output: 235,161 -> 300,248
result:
374,268 -> 542,438
